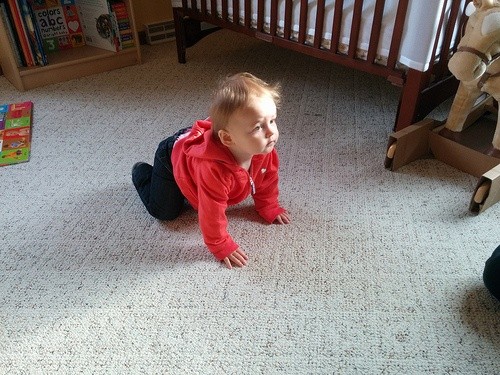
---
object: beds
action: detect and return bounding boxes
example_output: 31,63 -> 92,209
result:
171,0 -> 477,135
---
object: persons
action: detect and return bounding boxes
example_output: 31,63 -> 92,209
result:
131,73 -> 292,271
482,244 -> 500,302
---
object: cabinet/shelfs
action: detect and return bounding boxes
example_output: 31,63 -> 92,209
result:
143,18 -> 176,45
0,0 -> 142,92
